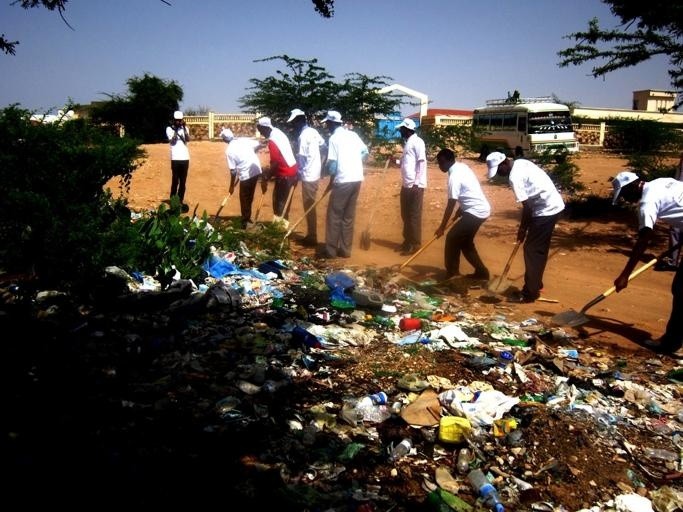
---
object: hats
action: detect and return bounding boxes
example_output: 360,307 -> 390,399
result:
174,111 -> 183,120
486,152 -> 506,178
221,129 -> 233,141
258,117 -> 274,129
321,111 -> 342,123
286,109 -> 305,122
611,172 -> 639,206
395,118 -> 416,131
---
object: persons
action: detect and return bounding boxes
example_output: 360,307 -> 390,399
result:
257,118 -> 296,220
316,109 -> 368,259
220,127 -> 264,228
388,118 -> 428,254
282,108 -> 326,247
611,171 -> 683,352
435,149 -> 492,279
484,151 -> 566,300
166,111 -> 191,201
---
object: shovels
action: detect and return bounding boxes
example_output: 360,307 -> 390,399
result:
551,243 -> 679,327
360,160 -> 389,250
488,240 -> 520,293
272,185 -> 294,229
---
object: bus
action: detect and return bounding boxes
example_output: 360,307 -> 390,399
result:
469,95 -> 579,163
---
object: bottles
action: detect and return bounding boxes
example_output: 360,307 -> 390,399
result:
456,445 -> 471,475
466,468 -> 505,511
383,436 -> 414,465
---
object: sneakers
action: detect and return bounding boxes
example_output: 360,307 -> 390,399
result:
467,270 -> 490,279
645,339 -> 682,358
297,235 -> 317,243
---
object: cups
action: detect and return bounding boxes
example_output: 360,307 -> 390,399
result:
363,392 -> 387,410
399,317 -> 422,330
